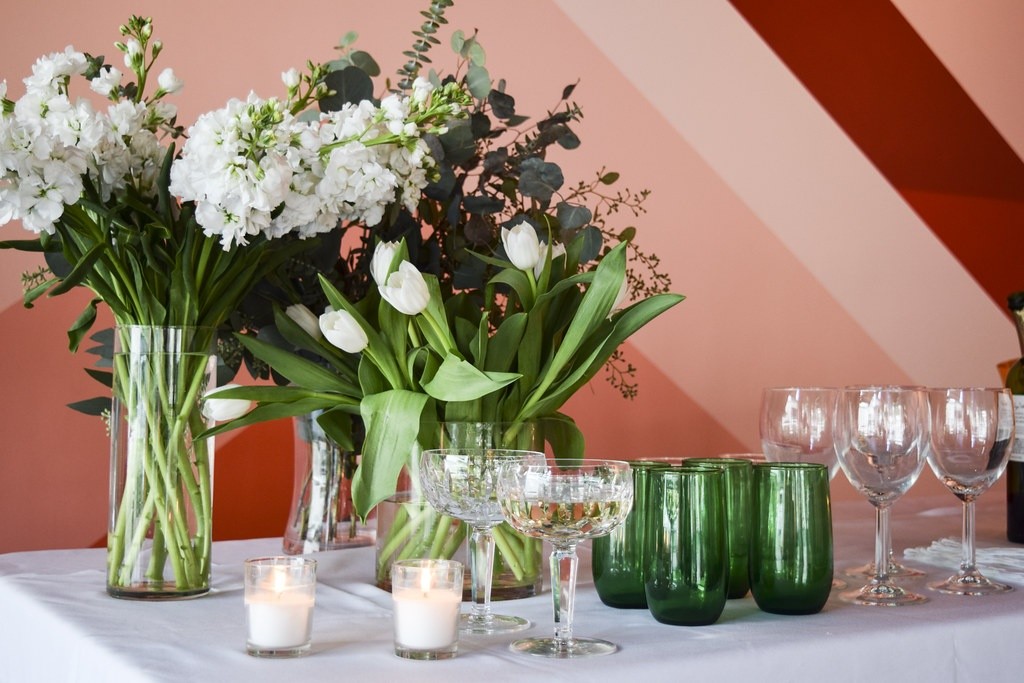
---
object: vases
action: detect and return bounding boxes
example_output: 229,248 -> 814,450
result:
370,424 -> 543,601
103,324 -> 221,600
278,414 -> 378,556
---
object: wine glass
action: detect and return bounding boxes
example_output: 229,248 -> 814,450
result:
758,386 -> 840,482
420,449 -> 548,636
844,506 -> 925,582
921,388 -> 1017,598
832,385 -> 929,607
497,459 -> 634,660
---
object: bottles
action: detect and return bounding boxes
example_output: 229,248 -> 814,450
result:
1004,291 -> 1024,551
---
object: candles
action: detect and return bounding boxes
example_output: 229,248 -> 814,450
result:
390,559 -> 465,660
243,560 -> 316,656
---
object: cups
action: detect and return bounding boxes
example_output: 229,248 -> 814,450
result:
591,461 -> 671,610
245,556 -> 317,660
750,463 -> 835,616
391,559 -> 465,660
634,468 -> 730,627
682,458 -> 755,598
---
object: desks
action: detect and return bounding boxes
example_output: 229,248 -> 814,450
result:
1,498 -> 1021,682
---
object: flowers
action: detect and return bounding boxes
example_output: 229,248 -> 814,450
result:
201,216 -> 538,424
0,15 -> 468,253
